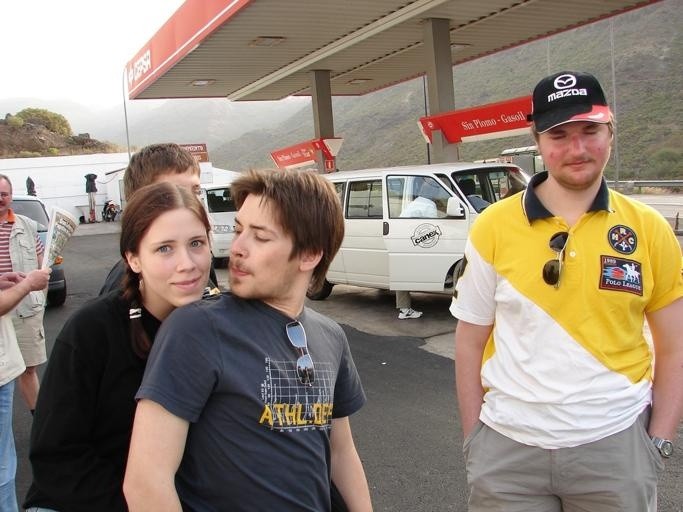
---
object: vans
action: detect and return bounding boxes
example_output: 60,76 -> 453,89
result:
306,161 -> 533,299
196,184 -> 242,269
9,195 -> 65,304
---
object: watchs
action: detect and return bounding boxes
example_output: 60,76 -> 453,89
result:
646,433 -> 676,459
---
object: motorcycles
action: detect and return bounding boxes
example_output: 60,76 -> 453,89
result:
101,200 -> 117,221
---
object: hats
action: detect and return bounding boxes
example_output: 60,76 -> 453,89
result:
527,71 -> 610,134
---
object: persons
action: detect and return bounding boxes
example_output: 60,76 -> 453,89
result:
23,181 -> 214,512
97,143 -> 219,296
123,166 -> 375,512
0,175 -> 52,417
449,70 -> 683,512
503,171 -> 526,200
0,269 -> 52,512
393,182 -> 439,319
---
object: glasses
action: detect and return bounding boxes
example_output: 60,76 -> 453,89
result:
285,319 -> 315,387
543,232 -> 570,288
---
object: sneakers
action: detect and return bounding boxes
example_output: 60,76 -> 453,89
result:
398,308 -> 423,320
